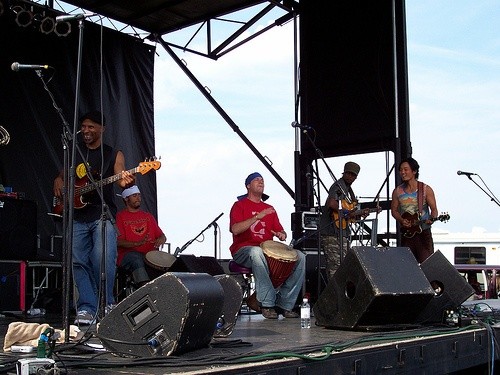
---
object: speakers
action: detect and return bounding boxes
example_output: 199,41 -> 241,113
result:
0,197 -> 39,261
214,273 -> 246,337
312,246 -> 435,330
94,272 -> 225,357
416,249 -> 476,323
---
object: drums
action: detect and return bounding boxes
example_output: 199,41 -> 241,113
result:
144,249 -> 176,281
245,240 -> 299,314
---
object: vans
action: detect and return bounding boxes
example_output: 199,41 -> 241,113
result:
452,264 -> 500,300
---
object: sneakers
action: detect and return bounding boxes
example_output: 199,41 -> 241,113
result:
74,310 -> 96,324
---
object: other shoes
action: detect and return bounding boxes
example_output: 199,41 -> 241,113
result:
275,306 -> 299,318
261,308 -> 277,319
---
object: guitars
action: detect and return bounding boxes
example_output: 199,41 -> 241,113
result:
331,198 -> 382,232
400,210 -> 450,238
52,156 -> 161,214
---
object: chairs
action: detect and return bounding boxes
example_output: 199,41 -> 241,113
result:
229,263 -> 260,315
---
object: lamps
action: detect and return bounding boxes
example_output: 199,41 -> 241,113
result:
7,2 -> 33,28
54,21 -> 74,38
33,13 -> 54,35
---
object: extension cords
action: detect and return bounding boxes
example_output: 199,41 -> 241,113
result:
14,357 -> 57,375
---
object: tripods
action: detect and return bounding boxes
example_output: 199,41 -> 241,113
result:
37,22 -> 108,353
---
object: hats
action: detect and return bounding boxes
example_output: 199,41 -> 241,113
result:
79,111 -> 106,126
344,162 -> 360,176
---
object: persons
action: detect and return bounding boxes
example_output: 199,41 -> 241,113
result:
391,157 -> 438,264
229,172 -> 306,319
54,111 -> 133,324
115,184 -> 166,287
320,162 -> 370,284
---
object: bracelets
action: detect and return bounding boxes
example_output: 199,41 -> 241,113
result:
255,216 -> 258,220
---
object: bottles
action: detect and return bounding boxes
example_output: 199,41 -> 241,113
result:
37,335 -> 46,358
300,298 -> 311,329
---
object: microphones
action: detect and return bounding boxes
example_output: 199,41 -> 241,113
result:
291,122 -> 311,129
11,62 -> 49,70
457,171 -> 476,175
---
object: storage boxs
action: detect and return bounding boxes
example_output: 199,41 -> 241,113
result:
290,231 -> 319,250
291,211 -> 322,230
302,249 -> 324,303
